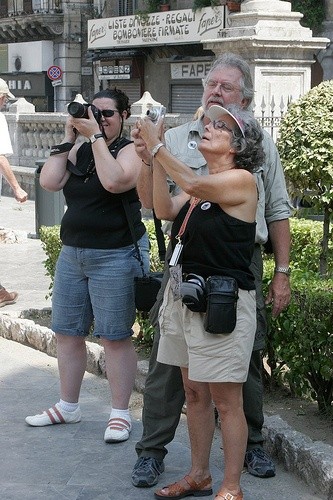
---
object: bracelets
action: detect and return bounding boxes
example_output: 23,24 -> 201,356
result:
142,159 -> 151,166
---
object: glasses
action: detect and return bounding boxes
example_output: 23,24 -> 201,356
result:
212,121 -> 233,133
101,110 -> 118,118
206,80 -> 244,93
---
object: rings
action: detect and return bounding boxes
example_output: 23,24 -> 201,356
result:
284,303 -> 288,305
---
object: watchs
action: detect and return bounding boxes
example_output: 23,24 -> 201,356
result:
275,266 -> 291,277
89,134 -> 105,144
151,143 -> 165,157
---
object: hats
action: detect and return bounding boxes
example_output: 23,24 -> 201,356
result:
0,78 -> 15,99
206,105 -> 245,138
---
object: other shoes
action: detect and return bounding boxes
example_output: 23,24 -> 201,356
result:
0,287 -> 17,307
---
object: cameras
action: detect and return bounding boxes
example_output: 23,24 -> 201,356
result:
67,101 -> 101,126
146,106 -> 165,124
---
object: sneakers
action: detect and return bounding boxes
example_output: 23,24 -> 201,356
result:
131,456 -> 165,487
103,414 -> 133,441
244,448 -> 275,477
25,404 -> 81,427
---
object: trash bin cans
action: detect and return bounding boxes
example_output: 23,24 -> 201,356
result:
35,161 -> 64,239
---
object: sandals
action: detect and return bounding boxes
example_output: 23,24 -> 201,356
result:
216,486 -> 244,500
154,473 -> 213,500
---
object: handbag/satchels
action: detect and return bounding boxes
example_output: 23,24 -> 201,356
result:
133,271 -> 164,311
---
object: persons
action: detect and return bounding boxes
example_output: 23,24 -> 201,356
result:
131,55 -> 293,487
24,87 -> 151,444
139,104 -> 267,500
0,78 -> 28,307
